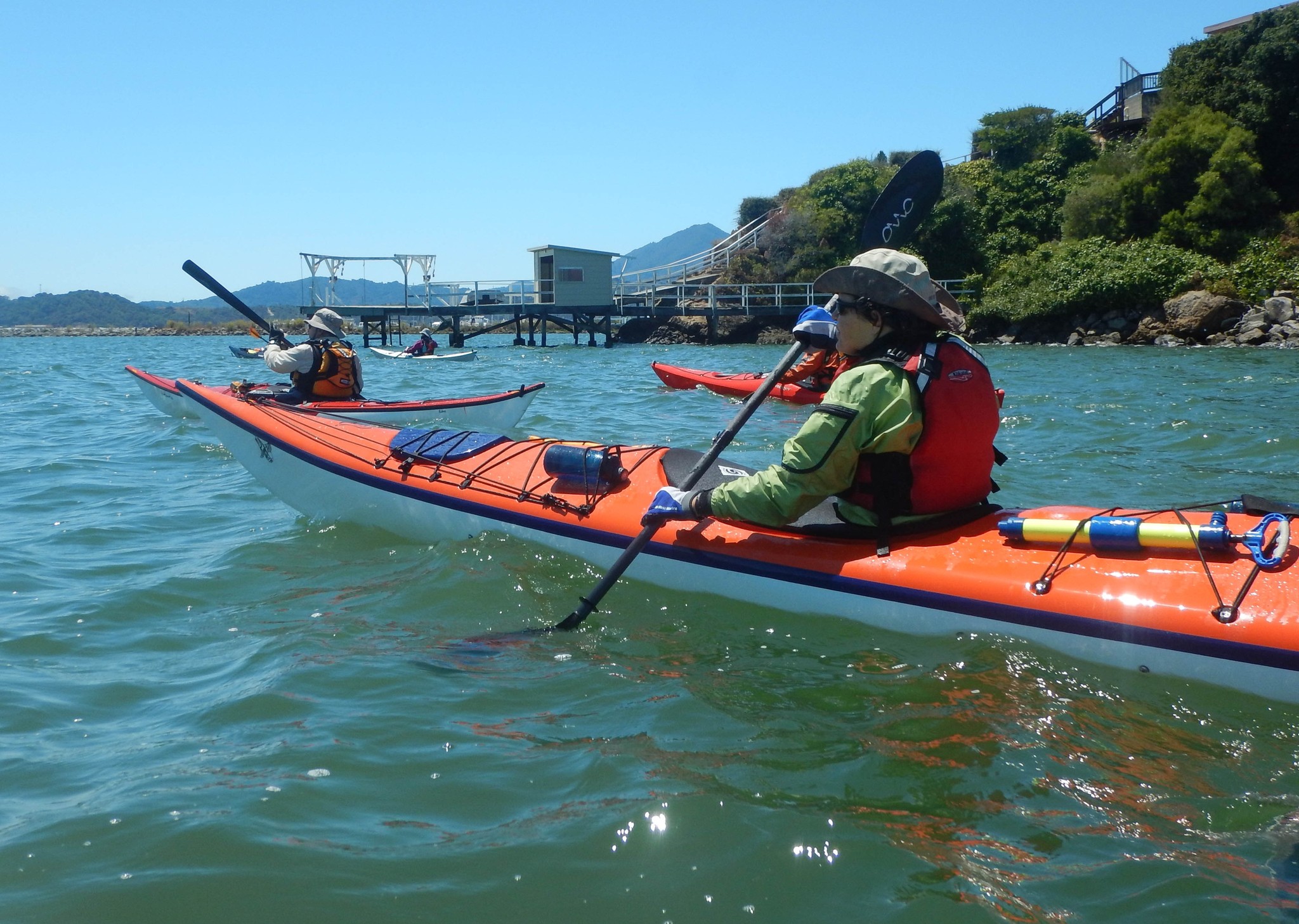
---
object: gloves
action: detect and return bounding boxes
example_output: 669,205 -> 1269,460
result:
269,321 -> 284,345
792,306 -> 838,349
640,486 -> 714,527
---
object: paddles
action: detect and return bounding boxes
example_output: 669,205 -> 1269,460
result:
393,317 -> 452,360
250,327 -> 271,344
422,148 -> 944,676
182,259 -> 369,400
741,391 -> 755,402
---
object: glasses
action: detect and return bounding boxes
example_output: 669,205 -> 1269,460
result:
308,324 -> 312,329
836,298 -> 856,315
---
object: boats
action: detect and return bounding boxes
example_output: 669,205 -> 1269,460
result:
368,347 -> 478,362
173,376 -> 1298,708
123,364 -> 546,430
651,359 -> 1006,410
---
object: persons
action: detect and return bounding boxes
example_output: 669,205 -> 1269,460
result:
254,308 -> 364,399
404,328 -> 438,356
641,248 -> 1009,556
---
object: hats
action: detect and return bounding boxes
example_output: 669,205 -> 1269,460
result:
304,308 -> 346,338
812,248 -> 966,331
279,328 -> 287,338
419,328 -> 430,337
